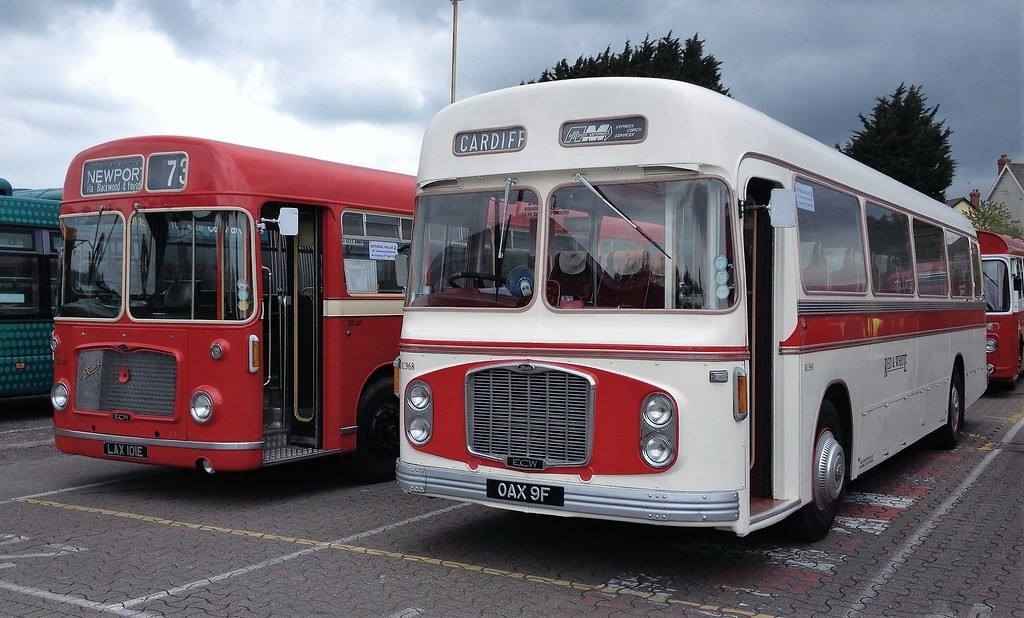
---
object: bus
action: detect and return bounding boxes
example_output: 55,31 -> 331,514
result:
976,229 -> 1024,390
0,176 -> 322,413
391,73 -> 987,546
887,260 -> 974,295
51,134 -> 665,484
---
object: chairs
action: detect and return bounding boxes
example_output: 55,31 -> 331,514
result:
649,254 -> 680,308
874,254 -> 910,293
822,247 -> 860,293
596,250 -> 651,309
151,283 -> 199,318
545,250 -> 594,309
800,241 -> 831,291
854,251 -> 881,292
